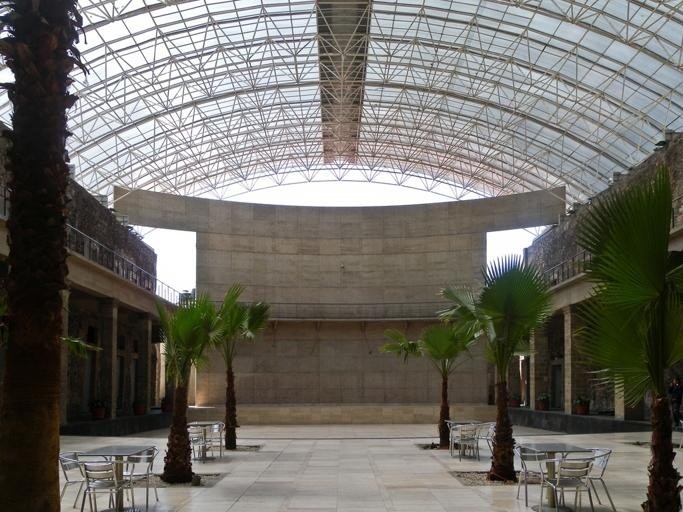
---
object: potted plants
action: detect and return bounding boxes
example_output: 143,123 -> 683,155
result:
534,391 -> 550,411
88,397 -> 107,420
509,391 -> 522,406
572,394 -> 589,414
160,395 -> 171,411
129,396 -> 148,415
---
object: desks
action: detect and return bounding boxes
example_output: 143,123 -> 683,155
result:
521,443 -> 590,511
76,445 -> 153,512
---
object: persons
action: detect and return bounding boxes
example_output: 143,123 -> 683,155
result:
668,374 -> 682,427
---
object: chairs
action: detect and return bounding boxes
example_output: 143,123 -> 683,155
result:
123,445 -> 161,501
57,447 -> 110,508
186,418 -> 225,460
76,458 -> 134,512
536,458 -> 593,511
445,415 -> 496,464
582,445 -> 617,512
513,443 -> 546,508
